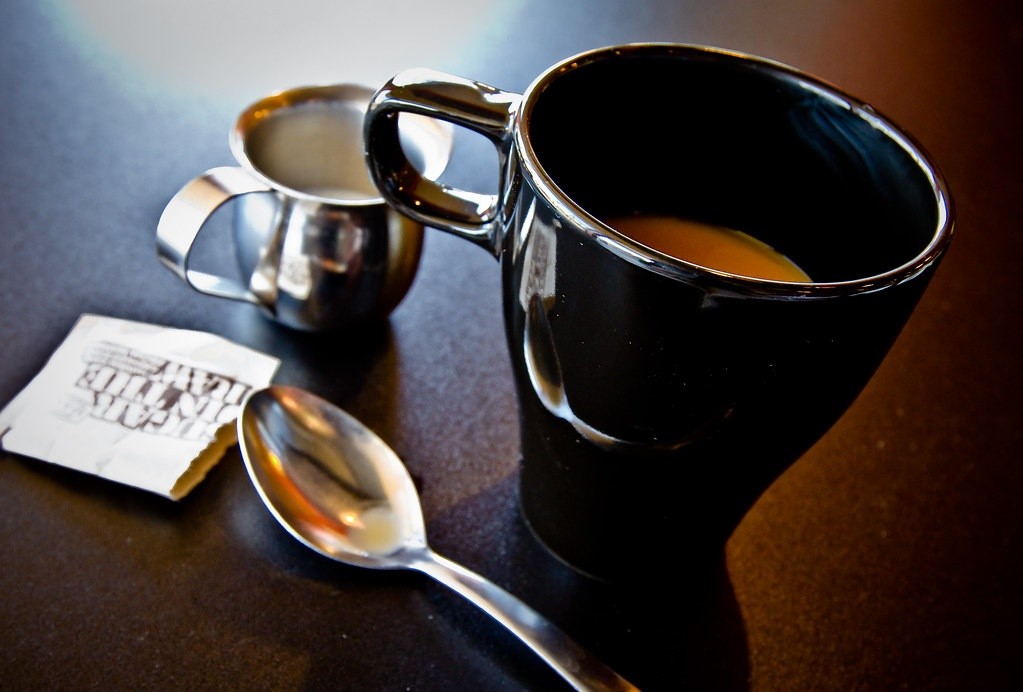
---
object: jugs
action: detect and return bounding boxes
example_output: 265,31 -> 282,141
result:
155,81 -> 455,331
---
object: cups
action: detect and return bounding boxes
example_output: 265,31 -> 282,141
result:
363,43 -> 955,583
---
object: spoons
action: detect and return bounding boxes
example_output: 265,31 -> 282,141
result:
237,384 -> 640,692
